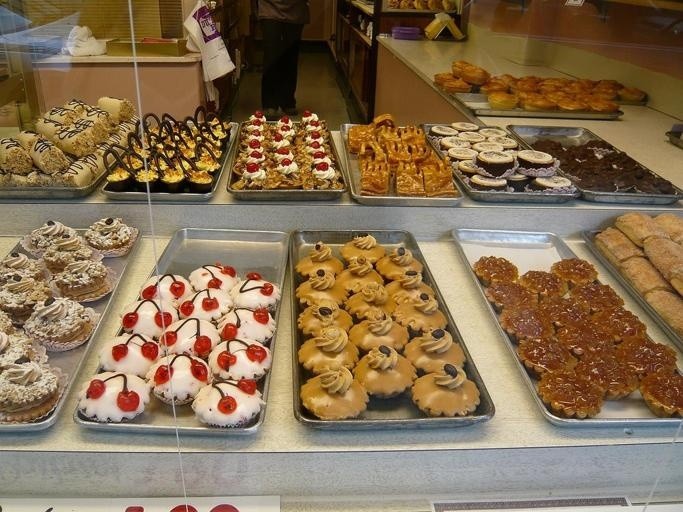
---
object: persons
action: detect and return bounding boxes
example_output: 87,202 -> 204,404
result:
256,0 -> 311,121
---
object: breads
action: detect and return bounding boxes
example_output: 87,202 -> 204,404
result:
348,310 -> 409,351
98,333 -> 164,379
191,378 -> 265,427
392,293 -> 448,335
348,114 -> 458,198
216,307 -> 276,344
295,241 -> 344,280
120,299 -> 179,339
298,303 -> 354,338
473,256 -> 683,419
0,217 -> 136,299
146,353 -> 210,406
352,345 -> 418,399
594,212 -> 683,339
430,122 -> 571,191
78,371 -> 151,423
159,318 -> 221,360
232,110 -> 343,190
296,269 -> 349,307
387,1 -> 454,12
335,256 -> 384,293
403,328 -> 466,374
0,96 -> 140,188
297,326 -> 359,374
434,60 -> 646,113
230,272 -> 280,310
532,139 -> 675,195
1,299 -> 97,422
207,338 -> 273,381
140,273 -> 195,305
412,363 -> 480,417
344,281 -> 395,320
103,105 -> 232,191
189,262 -> 237,293
300,366 -> 369,419
338,233 -> 386,264
384,270 -> 435,297
376,247 -> 423,281
178,288 -> 233,323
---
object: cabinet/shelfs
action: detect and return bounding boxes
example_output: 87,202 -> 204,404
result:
347,0 -> 461,121
373,34 -> 475,123
33,39 -> 208,129
335,0 -> 351,80
